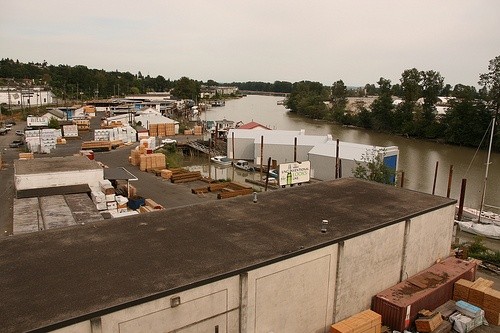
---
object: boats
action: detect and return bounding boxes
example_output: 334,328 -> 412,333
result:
234,158 -> 254,171
268,168 -> 279,177
211,155 -> 232,166
262,177 -> 278,187
455,204 -> 500,226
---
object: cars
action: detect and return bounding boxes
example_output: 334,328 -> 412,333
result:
1,115 -> 35,148
162,138 -> 176,145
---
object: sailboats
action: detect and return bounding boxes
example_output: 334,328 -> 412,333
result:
452,117 -> 500,242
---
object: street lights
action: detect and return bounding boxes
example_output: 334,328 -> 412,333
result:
126,177 -> 139,200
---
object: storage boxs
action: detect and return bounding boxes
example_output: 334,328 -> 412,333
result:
18,104 -> 203,221
329,255 -> 500,333
